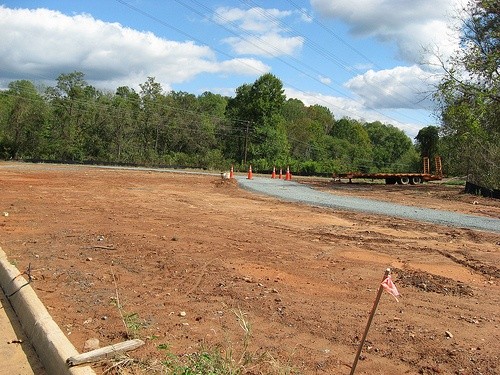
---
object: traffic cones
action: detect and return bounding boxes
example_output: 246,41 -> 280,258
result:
284,166 -> 291,181
270,165 -> 276,179
229,165 -> 233,178
245,164 -> 254,179
279,168 -> 283,179
